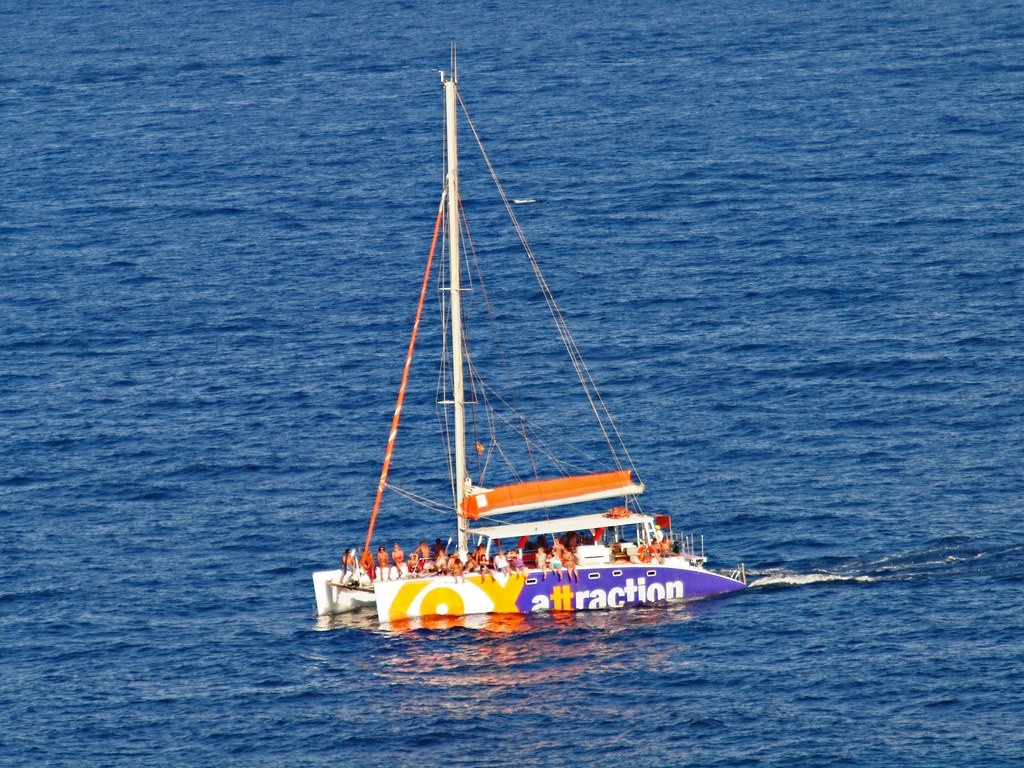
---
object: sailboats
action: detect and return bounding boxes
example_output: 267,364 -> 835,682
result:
306,46 -> 746,617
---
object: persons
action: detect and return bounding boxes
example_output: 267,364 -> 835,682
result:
408,524 -> 672,584
377,546 -> 392,581
391,544 -> 404,579
339,548 -> 355,584
362,548 -> 376,582
